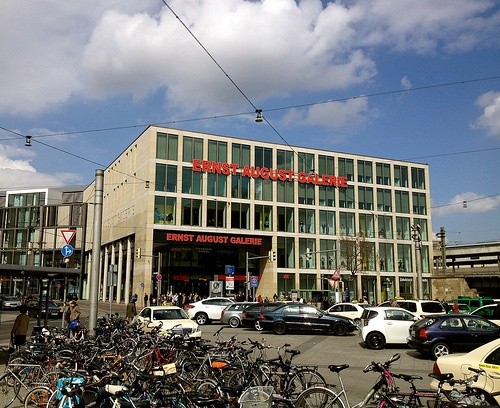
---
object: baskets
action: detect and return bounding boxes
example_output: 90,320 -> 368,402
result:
238,386 -> 274,408
153,363 -> 176,376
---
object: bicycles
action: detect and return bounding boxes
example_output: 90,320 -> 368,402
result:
1,312 -> 500,408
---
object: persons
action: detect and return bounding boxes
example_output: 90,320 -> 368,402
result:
150,293 -> 206,308
372,300 -> 375,307
300,221 -> 304,232
390,299 -> 400,307
442,300 -> 451,314
65,300 -> 81,334
144,293 -> 148,307
236,292 -> 308,305
360,298 -> 369,305
322,222 -> 325,234
453,302 -> 460,314
126,294 -> 138,322
154,207 -> 173,221
9,305 -> 30,355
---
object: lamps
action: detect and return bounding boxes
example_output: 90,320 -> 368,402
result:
255,109 -> 263,122
25,135 -> 31,146
463,200 -> 468,208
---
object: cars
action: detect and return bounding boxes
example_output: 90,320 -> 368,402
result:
240,301 -> 285,331
19,293 -> 78,319
220,302 -> 259,328
132,305 -> 202,343
188,296 -> 249,325
259,303 -> 355,336
358,307 -> 423,350
376,295 -> 447,319
1,295 -> 22,310
444,296 -> 500,327
406,314 -> 500,361
324,299 -> 373,330
429,338 -> 500,407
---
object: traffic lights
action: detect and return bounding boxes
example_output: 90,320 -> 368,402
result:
135,248 -> 142,260
270,250 -> 277,262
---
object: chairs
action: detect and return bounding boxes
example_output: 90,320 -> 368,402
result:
453,320 -> 459,327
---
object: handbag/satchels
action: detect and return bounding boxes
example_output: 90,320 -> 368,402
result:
69,319 -> 80,331
9,335 -> 14,353
